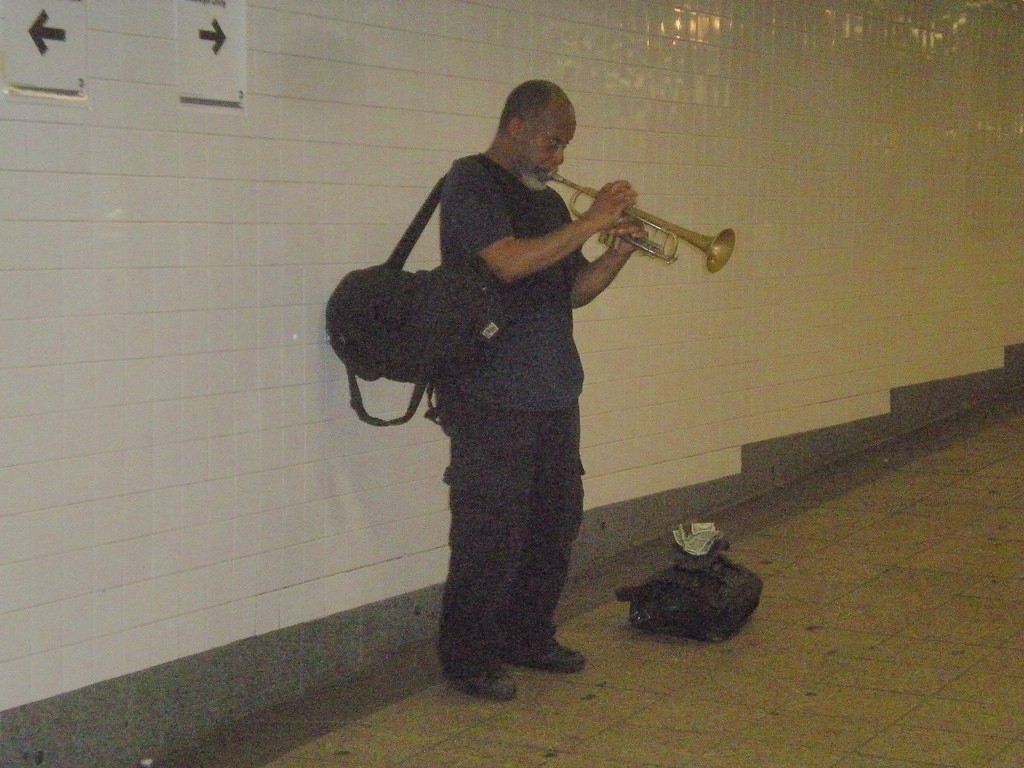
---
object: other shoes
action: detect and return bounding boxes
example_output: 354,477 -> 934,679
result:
504,640 -> 586,674
453,665 -> 518,702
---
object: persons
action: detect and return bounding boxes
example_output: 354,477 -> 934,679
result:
437,78 -> 650,700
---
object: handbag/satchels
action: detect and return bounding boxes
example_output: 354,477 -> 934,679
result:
615,539 -> 763,642
326,154 -> 518,426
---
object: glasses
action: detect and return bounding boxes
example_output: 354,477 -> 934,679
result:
522,119 -> 573,151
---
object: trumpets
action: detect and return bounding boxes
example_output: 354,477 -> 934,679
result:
549,167 -> 738,274
